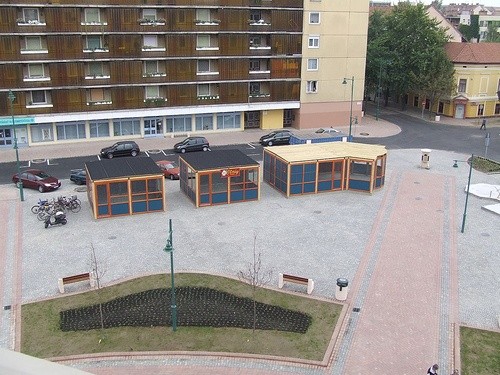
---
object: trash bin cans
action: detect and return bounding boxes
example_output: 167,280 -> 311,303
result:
335,277 -> 349,301
435,116 -> 441,121
421,149 -> 432,170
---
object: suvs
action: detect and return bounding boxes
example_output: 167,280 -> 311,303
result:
259,130 -> 294,146
100,140 -> 140,159
174,136 -> 210,154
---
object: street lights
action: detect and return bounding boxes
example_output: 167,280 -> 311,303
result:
163,219 -> 177,332
7,89 -> 25,202
342,76 -> 354,136
452,154 -> 474,234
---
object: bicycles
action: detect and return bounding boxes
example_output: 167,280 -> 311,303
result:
31,194 -> 82,223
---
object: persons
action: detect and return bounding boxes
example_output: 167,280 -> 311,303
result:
427,364 -> 439,375
480,117 -> 486,130
452,368 -> 460,375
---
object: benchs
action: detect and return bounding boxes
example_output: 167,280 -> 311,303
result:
278,273 -> 315,294
58,272 -> 95,294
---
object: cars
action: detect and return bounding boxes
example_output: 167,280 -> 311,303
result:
70,168 -> 88,185
12,169 -> 61,193
155,160 -> 180,180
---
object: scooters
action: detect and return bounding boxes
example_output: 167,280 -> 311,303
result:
43,210 -> 67,229
